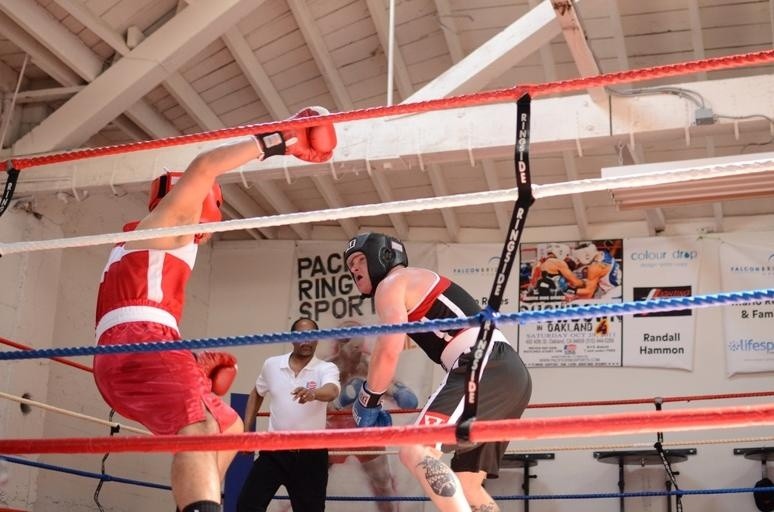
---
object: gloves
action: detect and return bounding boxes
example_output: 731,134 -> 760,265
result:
191,349 -> 239,397
387,378 -> 420,410
348,379 -> 394,429
248,103 -> 338,164
332,376 -> 364,412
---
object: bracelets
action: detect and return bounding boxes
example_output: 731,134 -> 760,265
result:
308,389 -> 316,401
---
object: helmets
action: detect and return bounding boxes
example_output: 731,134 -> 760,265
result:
341,231 -> 409,300
564,251 -> 581,266
146,166 -> 225,244
571,242 -> 599,266
545,243 -> 570,260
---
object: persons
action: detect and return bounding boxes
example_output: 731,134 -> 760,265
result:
521,252 -> 590,311
91,104 -> 338,512
318,319 -> 421,512
556,241 -> 622,302
342,229 -> 534,512
236,315 -> 342,512
